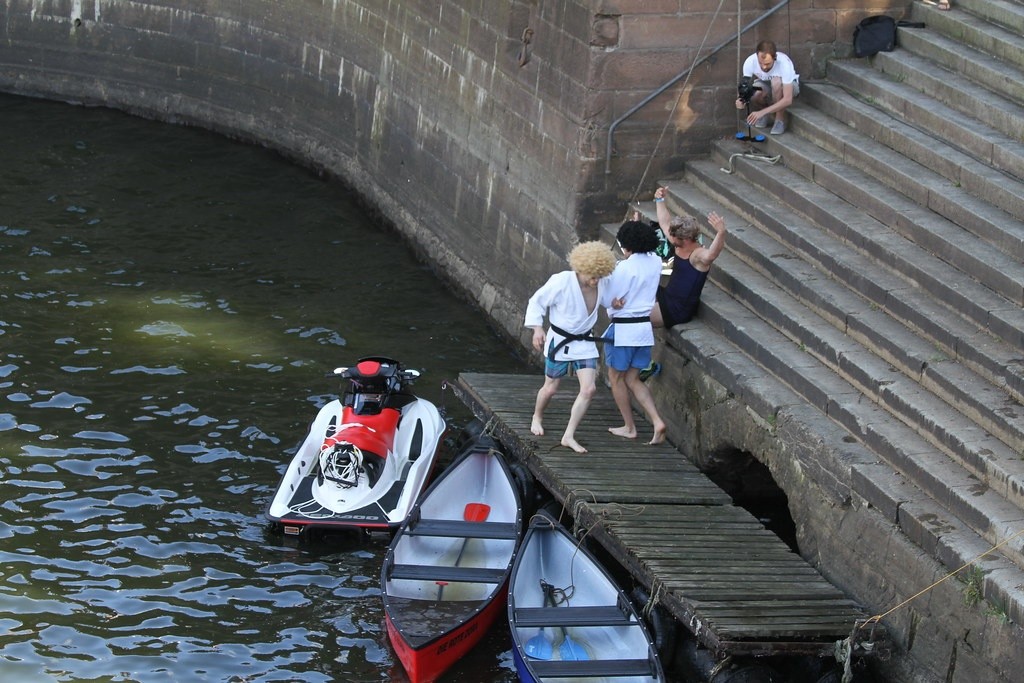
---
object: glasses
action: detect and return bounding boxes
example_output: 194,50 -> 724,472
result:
665,240 -> 685,250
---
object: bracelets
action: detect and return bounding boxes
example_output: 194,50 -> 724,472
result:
656,198 -> 664,202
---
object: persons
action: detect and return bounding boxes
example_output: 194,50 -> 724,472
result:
938,0 -> 950,11
649,187 -> 725,329
736,40 -> 800,135
600,221 -> 667,445
524,241 -> 627,452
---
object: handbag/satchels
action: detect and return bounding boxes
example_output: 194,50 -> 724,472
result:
853,15 -> 898,57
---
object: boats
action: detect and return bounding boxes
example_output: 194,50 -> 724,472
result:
507,509 -> 666,683
380,430 -> 521,683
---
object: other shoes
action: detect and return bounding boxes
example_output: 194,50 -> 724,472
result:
660,256 -> 676,276
755,115 -> 769,129
770,120 -> 789,135
638,359 -> 661,382
648,220 -> 675,259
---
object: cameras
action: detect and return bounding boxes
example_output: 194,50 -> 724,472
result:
738,75 -> 762,103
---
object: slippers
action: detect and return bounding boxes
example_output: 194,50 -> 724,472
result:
938,0 -> 951,11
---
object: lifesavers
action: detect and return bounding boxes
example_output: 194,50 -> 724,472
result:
509,463 -> 544,520
628,591 -> 674,666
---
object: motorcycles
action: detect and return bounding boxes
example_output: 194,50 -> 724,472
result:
264,356 -> 447,539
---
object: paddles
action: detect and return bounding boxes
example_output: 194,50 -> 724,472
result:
524,583 -> 553,660
454,503 -> 490,566
543,587 -> 590,661
435,581 -> 449,601
